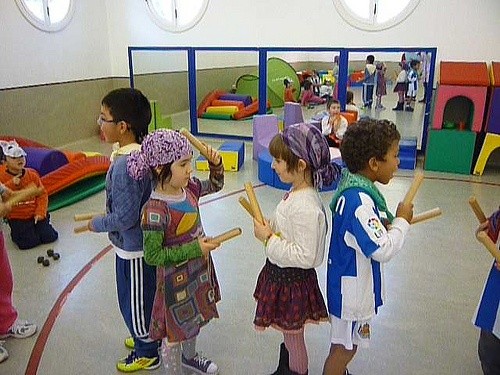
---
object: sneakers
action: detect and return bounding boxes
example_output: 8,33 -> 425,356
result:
126,336 -> 162,350
117,351 -> 162,373
0,341 -> 10,363
0,317 -> 38,339
182,351 -> 217,375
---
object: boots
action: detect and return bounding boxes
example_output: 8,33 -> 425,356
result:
393,101 -> 404,110
270,343 -> 308,375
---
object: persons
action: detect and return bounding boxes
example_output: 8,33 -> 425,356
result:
87,87 -> 160,372
252,122 -> 330,375
0,139 -> 59,250
320,99 -> 348,148
359,54 -> 376,109
322,115 -> 415,375
474,207 -> 500,375
405,59 -> 420,112
284,79 -> 297,102
331,55 -> 340,101
375,61 -> 387,110
419,51 -> 431,103
346,91 -> 360,122
310,69 -> 322,96
300,82 -> 326,106
392,53 -> 408,111
125,128 -> 225,375
0,181 -> 38,363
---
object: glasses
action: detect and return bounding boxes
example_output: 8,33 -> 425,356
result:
97,115 -> 113,122
